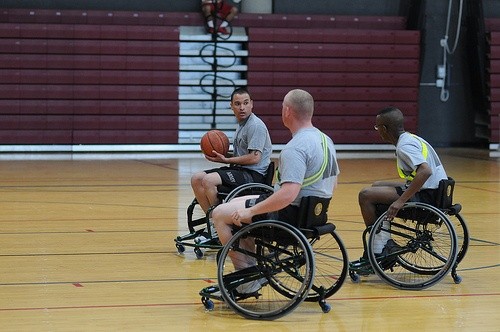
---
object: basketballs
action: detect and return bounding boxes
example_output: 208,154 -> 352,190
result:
200,130 -> 230,158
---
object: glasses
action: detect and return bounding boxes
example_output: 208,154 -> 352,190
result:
374,124 -> 388,130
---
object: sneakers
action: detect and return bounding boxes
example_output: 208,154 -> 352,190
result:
355,239 -> 402,275
194,224 -> 233,245
212,272 -> 268,299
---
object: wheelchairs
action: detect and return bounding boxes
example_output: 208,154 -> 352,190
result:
198,196 -> 349,323
173,160 -> 275,258
348,176 -> 469,291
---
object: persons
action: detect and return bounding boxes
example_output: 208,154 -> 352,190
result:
213,89 -> 340,299
358,106 -> 450,260
202,0 -> 238,33
191,88 -> 272,244
